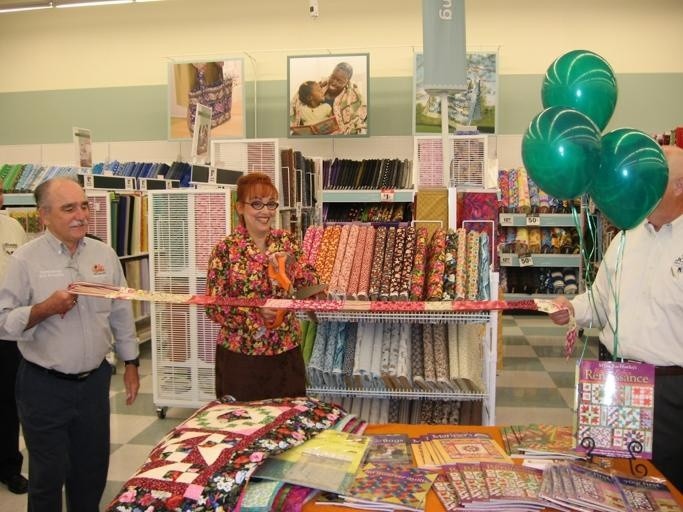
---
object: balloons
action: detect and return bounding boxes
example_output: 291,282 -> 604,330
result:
521,105 -> 603,200
541,50 -> 619,145
586,127 -> 669,230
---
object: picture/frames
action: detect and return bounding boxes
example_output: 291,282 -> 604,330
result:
167,57 -> 244,142
286,54 -> 368,138
412,51 -> 498,139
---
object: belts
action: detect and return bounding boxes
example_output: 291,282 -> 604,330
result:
22,360 -> 106,381
599,348 -> 683,377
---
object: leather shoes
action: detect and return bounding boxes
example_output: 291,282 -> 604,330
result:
0,473 -> 30,494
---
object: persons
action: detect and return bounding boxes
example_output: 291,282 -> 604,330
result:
549,144 -> 683,499
0,177 -> 140,512
298,80 -> 334,136
290,62 -> 366,135
0,180 -> 29,494
206,173 -> 321,401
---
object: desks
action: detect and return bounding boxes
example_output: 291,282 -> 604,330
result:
285,422 -> 683,512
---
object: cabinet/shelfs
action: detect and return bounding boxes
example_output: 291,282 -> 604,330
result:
414,136 -> 497,274
321,186 -> 414,234
293,273 -> 500,426
497,207 -> 585,311
150,189 -> 241,417
3,189 -> 150,365
213,139 -> 321,245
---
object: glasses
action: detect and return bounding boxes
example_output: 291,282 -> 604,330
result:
239,200 -> 279,210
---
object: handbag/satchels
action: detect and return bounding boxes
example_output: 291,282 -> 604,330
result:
186,79 -> 233,136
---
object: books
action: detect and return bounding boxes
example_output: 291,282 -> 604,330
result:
499,422 -> 592,460
540,460 -> 681,512
409,432 -> 514,468
429,462 -> 545,512
240,430 -> 439,512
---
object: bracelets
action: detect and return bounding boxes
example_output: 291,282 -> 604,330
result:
123,357 -> 140,367
